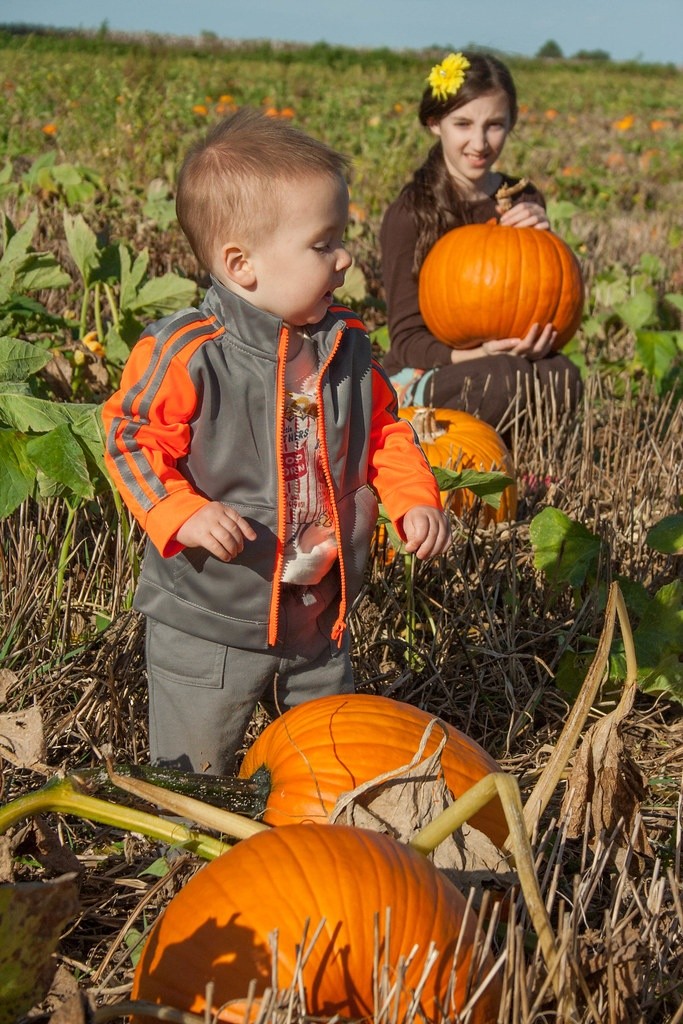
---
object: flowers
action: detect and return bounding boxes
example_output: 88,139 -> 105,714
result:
424,52 -> 471,101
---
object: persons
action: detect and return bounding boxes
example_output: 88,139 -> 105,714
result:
378,53 -> 583,451
98,107 -> 455,775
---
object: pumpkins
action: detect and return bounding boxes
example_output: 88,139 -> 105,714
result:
418,174 -> 585,354
50,694 -> 511,1024
369,406 -> 516,564
